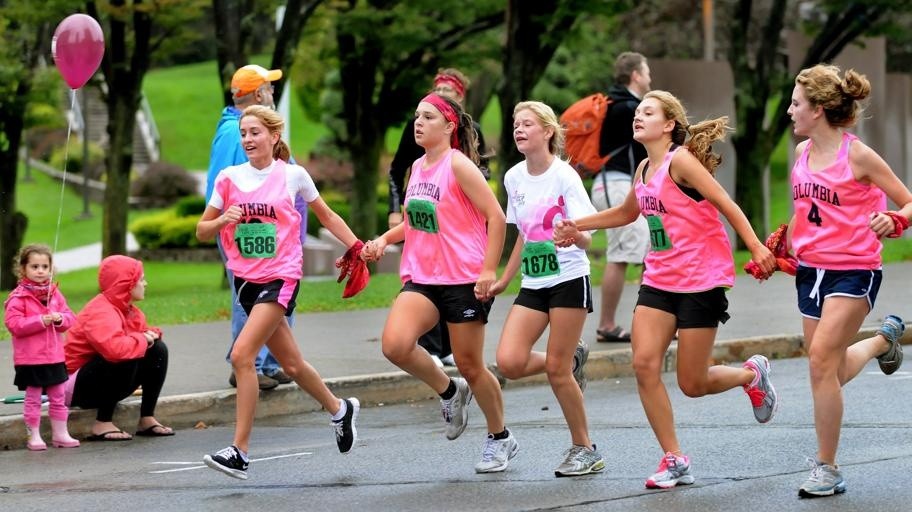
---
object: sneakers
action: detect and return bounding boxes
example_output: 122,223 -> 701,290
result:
228,370 -> 295,390
645,452 -> 694,488
475,428 -> 520,474
202,445 -> 251,480
440,377 -> 474,440
798,464 -> 846,498
742,354 -> 778,423
554,444 -> 605,477
875,314 -> 906,375
565,337 -> 590,393
331,397 -> 360,454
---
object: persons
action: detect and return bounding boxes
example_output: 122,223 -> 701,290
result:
196,104 -> 378,481
361,90 -> 520,474
387,67 -> 494,369
590,50 -> 654,345
64,254 -> 176,441
550,89 -> 776,489
757,61 -> 911,500
469,99 -> 608,476
202,64 -> 309,393
2,244 -> 81,450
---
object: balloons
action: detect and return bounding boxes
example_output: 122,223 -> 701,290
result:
52,14 -> 106,91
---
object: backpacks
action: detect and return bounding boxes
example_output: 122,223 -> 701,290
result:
557,91 -> 646,175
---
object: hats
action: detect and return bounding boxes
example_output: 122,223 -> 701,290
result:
230,64 -> 283,98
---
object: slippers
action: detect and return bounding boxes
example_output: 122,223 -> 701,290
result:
88,429 -> 133,440
135,425 -> 175,437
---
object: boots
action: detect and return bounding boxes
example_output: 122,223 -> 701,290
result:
23,414 -> 48,451
50,411 -> 81,448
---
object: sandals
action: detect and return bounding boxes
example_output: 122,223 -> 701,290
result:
597,326 -> 637,343
